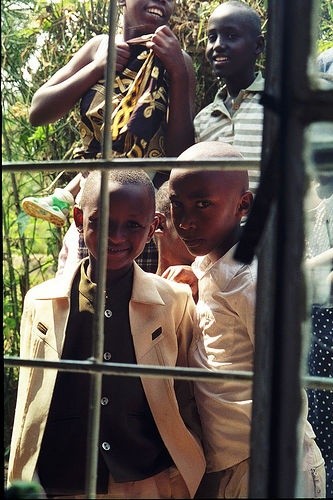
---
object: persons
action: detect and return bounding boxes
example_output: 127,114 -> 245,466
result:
193,0 -> 265,227
302,46 -> 333,500
23,0 -> 196,274
7,169 -> 206,499
161,141 -> 326,499
151,180 -> 196,277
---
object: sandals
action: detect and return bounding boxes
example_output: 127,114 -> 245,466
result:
22,195 -> 70,227
